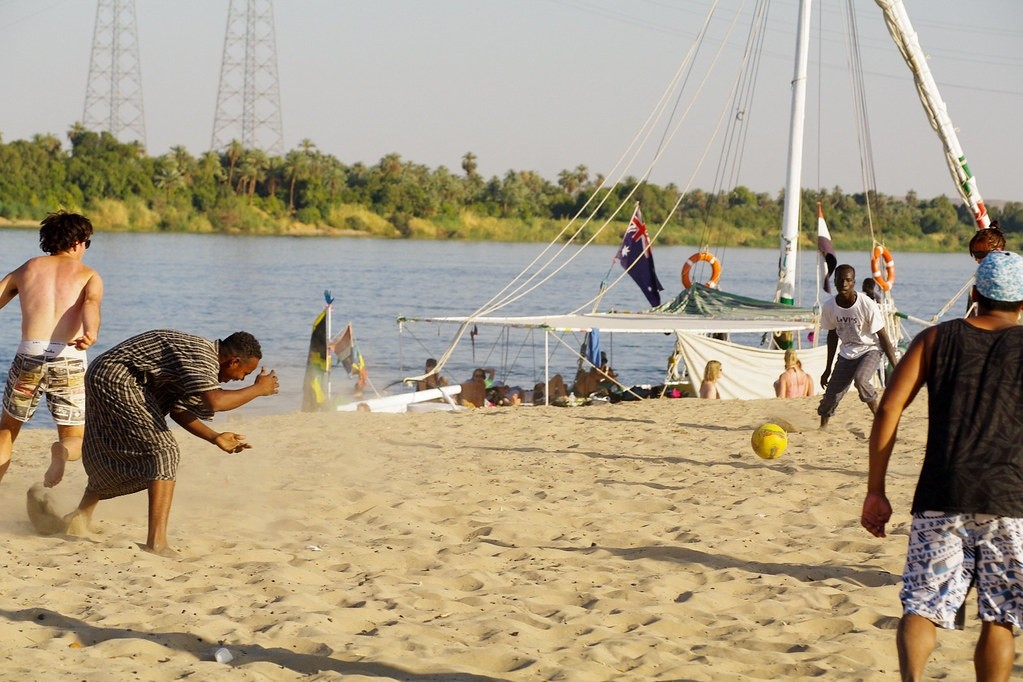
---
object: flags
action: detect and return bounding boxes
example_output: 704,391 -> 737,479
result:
816,201 -> 838,293
615,207 -> 665,308
302,307 -> 366,413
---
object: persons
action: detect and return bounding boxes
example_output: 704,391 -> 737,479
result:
966,229 -> 1006,318
862,278 -> 881,303
356,402 -> 370,413
416,351 -> 622,407
700,360 -> 722,399
774,349 -> 814,398
818,265 -> 898,431
861,252 -> 1023,682
0,210 -> 104,487
63,330 -> 279,559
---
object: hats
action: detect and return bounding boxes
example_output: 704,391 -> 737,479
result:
976,251 -> 1023,302
600,351 -> 608,364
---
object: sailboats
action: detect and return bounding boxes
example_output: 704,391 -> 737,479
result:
332,0 -> 992,413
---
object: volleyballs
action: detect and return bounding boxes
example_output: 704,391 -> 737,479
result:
751,424 -> 788,458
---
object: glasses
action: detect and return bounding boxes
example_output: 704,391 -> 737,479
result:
79,239 -> 92,249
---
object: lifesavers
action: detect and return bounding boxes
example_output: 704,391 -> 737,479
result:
871,246 -> 895,291
682,253 -> 721,289
